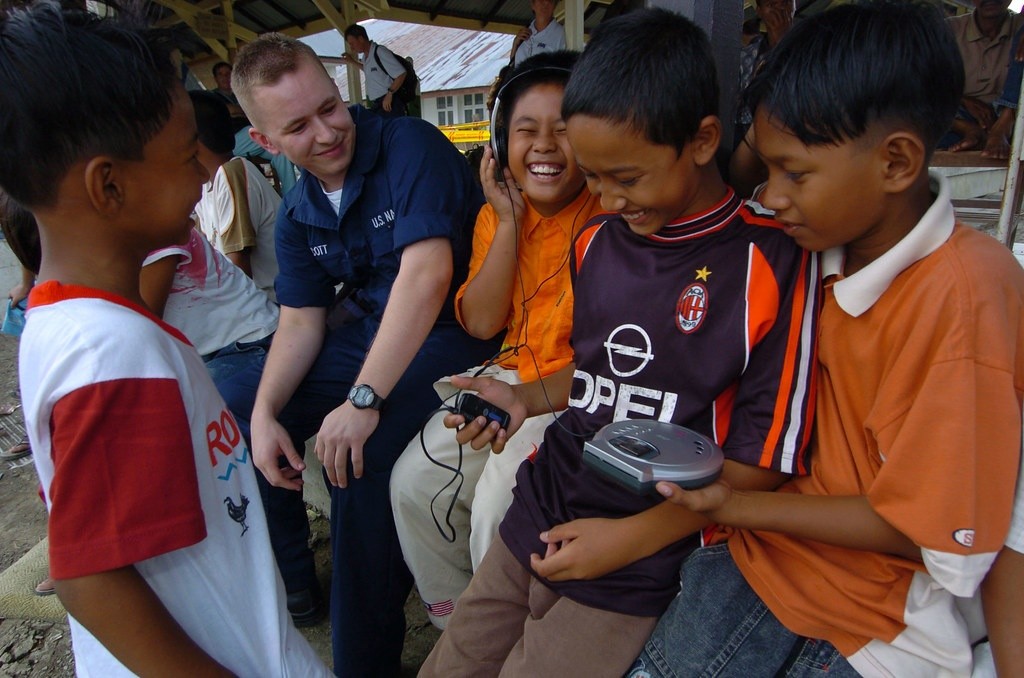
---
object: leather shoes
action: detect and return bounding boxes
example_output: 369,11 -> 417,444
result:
287,578 -> 325,627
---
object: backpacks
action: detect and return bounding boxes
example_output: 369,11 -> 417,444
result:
465,144 -> 484,161
374,45 -> 417,103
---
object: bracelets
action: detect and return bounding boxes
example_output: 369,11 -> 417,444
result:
388,88 -> 394,94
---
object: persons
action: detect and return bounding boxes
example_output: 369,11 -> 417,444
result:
0,0 -> 1024,678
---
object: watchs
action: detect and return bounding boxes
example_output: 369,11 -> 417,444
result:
347,384 -> 384,411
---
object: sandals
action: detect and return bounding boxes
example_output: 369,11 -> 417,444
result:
0,436 -> 32,460
34,576 -> 56,596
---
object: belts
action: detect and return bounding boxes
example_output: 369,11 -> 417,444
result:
372,94 -> 387,104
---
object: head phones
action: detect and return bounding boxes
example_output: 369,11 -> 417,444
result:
489,67 -> 573,170
